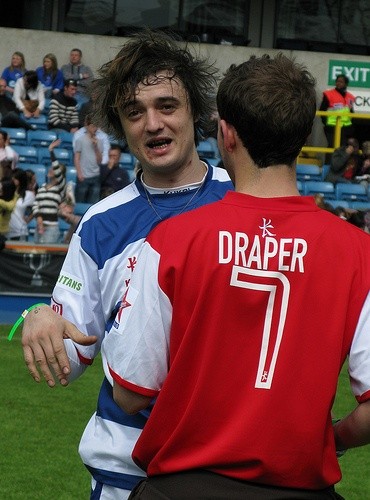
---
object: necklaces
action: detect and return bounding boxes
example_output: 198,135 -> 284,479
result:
142,166 -> 202,220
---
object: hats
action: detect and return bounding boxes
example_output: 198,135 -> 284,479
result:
64,79 -> 80,86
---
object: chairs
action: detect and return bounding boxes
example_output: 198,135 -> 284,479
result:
0,91 -> 370,243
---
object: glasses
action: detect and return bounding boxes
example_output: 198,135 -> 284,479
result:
69,80 -> 80,85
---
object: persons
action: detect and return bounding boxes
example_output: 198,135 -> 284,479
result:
320,75 -> 355,165
101,51 -> 370,500
327,136 -> 370,184
99,146 -> 129,200
316,194 -> 370,232
0,130 -> 80,242
0,49 -> 100,150
7,31 -> 233,500
75,113 -> 103,202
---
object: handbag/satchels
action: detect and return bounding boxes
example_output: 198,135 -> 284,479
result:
20,98 -> 39,114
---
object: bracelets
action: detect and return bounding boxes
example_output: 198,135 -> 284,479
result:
8,303 -> 48,341
